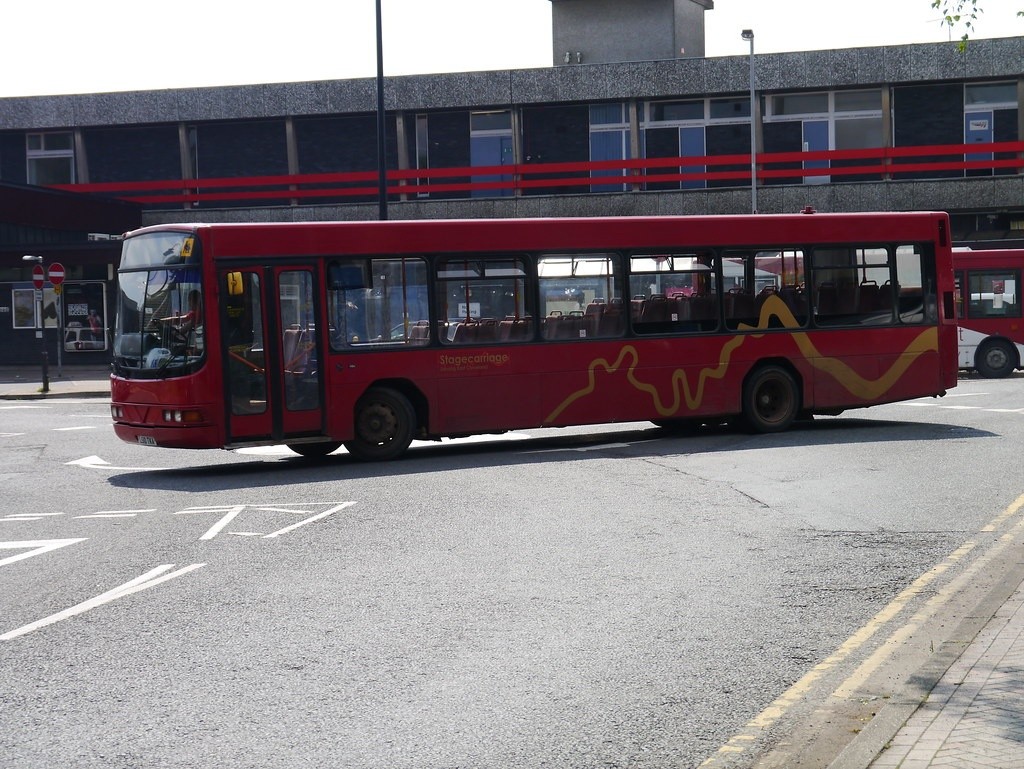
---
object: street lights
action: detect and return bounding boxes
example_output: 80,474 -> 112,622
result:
741,29 -> 758,213
23,255 -> 51,391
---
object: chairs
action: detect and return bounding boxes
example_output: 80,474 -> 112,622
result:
970,299 -> 1021,317
408,280 -> 902,344
283,323 -> 336,377
956,302 -> 962,318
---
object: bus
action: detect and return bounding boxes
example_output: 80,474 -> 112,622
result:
652,248 -> 1024,380
106,205 -> 959,465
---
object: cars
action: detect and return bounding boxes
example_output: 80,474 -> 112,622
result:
63,324 -> 110,354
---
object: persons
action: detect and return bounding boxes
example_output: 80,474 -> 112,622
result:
85,307 -> 104,349
150,289 -> 203,335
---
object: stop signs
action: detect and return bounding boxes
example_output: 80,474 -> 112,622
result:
33,265 -> 45,290
48,263 -> 66,286
